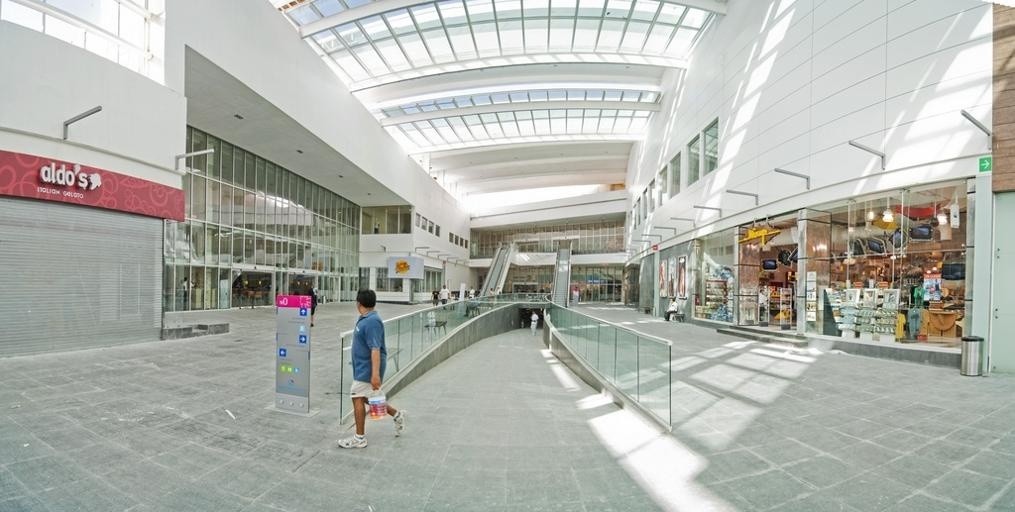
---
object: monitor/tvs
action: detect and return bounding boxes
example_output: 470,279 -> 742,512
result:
940,263 -> 966,280
888,227 -> 909,250
763,259 -> 777,270
867,236 -> 885,256
911,224 -> 931,240
845,239 -> 864,256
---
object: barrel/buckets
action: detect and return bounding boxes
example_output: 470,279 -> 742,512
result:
367,388 -> 388,421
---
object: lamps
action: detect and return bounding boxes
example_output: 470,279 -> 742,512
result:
867,200 -> 875,221
936,188 -> 948,227
881,195 -> 894,223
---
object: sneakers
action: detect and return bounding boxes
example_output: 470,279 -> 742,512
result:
338,435 -> 367,448
393,410 -> 406,436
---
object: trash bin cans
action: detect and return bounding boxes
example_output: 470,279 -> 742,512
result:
960,336 -> 984,376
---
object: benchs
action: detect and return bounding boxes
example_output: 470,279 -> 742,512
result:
670,313 -> 686,322
640,307 -> 652,314
470,306 -> 481,317
425,321 -> 448,337
350,348 -> 402,371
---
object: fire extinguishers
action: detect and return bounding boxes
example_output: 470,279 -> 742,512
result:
695,293 -> 700,305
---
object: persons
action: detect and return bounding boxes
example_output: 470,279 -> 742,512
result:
337,289 -> 407,449
307,288 -> 318,326
489,287 -> 501,296
431,285 -> 451,310
531,311 -> 538,335
466,286 -> 480,317
663,298 -> 678,322
182,277 -> 194,303
573,287 -> 579,305
